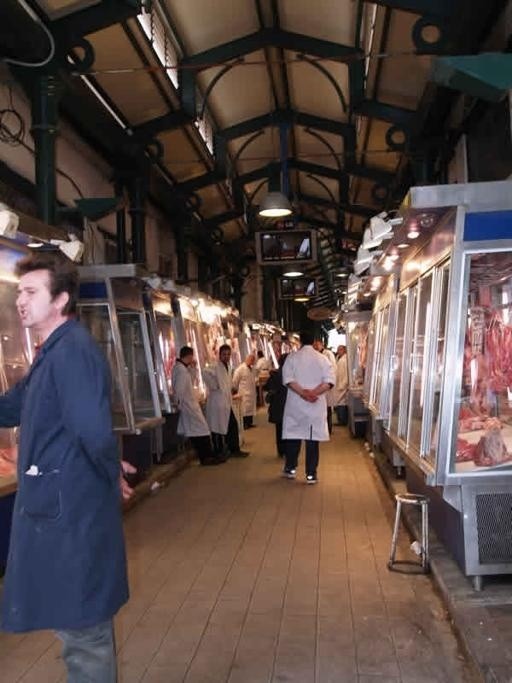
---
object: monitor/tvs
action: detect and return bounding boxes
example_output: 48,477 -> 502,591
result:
254,228 -> 318,266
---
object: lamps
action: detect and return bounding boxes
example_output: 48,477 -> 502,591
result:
257,78 -> 295,218
341,210 -> 420,313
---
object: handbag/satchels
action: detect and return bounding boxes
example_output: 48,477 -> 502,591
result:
264,390 -> 274,403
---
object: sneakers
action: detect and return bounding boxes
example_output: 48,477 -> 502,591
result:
230,450 -> 250,457
306,474 -> 318,484
282,466 -> 296,479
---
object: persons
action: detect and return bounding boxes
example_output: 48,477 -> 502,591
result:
2,247 -> 140,683
264,352 -> 289,459
232,354 -> 257,429
254,350 -> 273,405
313,338 -> 338,435
201,344 -> 250,462
172,345 -> 221,465
333,344 -> 348,426
280,327 -> 336,484
